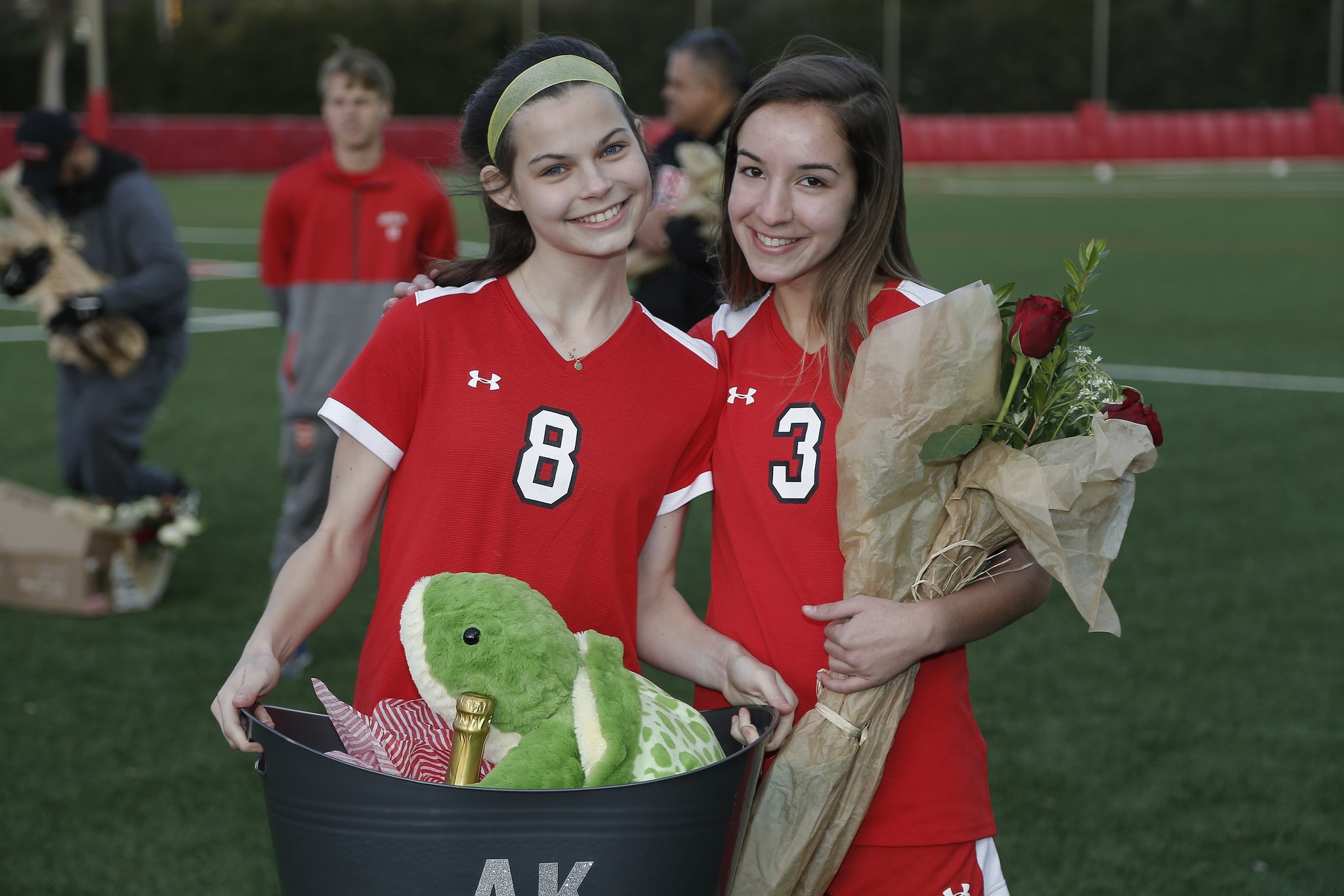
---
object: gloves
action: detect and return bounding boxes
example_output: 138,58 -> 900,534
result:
46,293 -> 104,334
2,245 -> 50,297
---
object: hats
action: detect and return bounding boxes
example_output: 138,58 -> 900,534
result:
11,112 -> 79,196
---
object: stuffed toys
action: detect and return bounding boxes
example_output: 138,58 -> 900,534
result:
398,569 -> 728,789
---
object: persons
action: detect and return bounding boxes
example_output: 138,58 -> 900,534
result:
254,38 -> 471,693
0,102 -> 212,528
619,30 -> 772,355
374,51 -> 1060,896
203,32 -> 800,896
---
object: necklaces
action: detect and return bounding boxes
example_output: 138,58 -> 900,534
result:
518,264 -> 631,371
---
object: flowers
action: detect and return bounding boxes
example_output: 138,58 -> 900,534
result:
715,222 -> 1162,896
